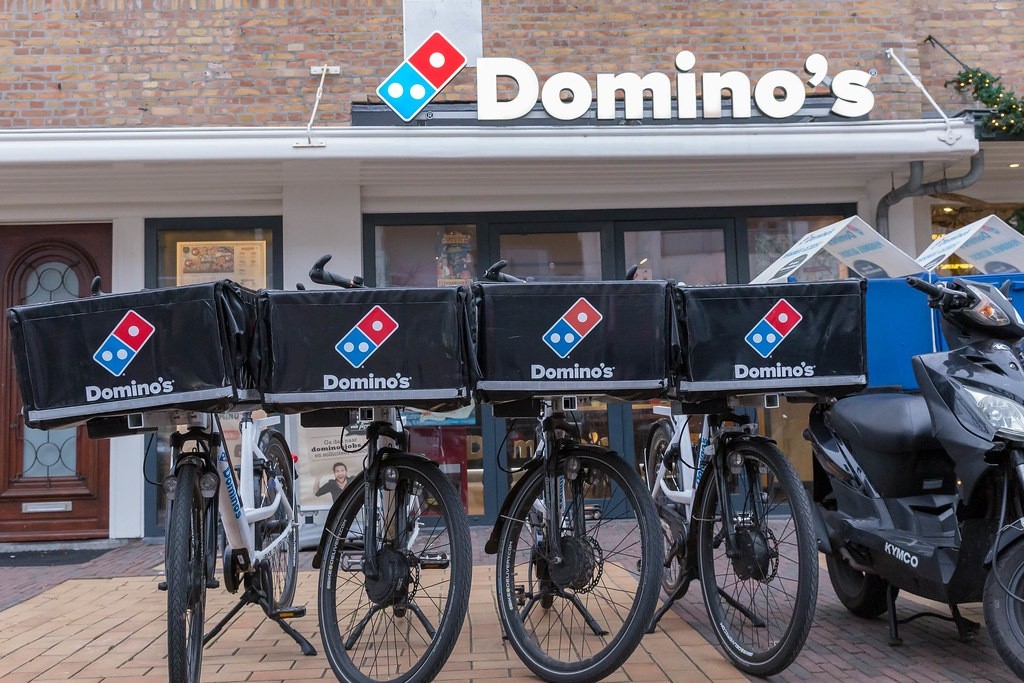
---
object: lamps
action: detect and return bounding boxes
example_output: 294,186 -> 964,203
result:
886,46 -> 975,151
291,63 -> 341,150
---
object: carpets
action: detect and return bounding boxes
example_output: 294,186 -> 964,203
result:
0,548 -> 113,566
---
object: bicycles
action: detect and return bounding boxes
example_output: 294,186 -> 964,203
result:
472,260 -> 681,683
645,278 -> 869,677
434,563 -> 487,683
7,276 -> 318,683
258,254 -> 474,683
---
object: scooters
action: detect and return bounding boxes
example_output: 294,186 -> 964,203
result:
802,271 -> 1024,683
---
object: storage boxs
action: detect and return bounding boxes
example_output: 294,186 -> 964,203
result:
787,271 -> 1024,391
471,279 -> 681,395
6,279 -> 265,432
672,278 -> 868,400
256,286 -> 481,417
